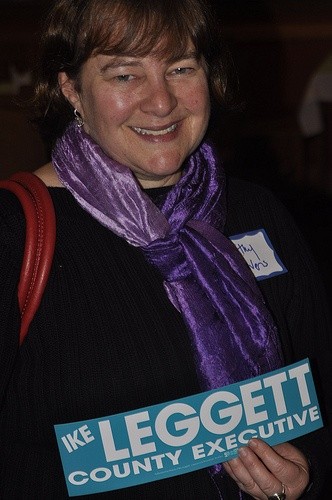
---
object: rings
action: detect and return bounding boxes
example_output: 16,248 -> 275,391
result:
267,484 -> 285,500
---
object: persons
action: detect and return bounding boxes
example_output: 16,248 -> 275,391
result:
1,1 -> 332,500
